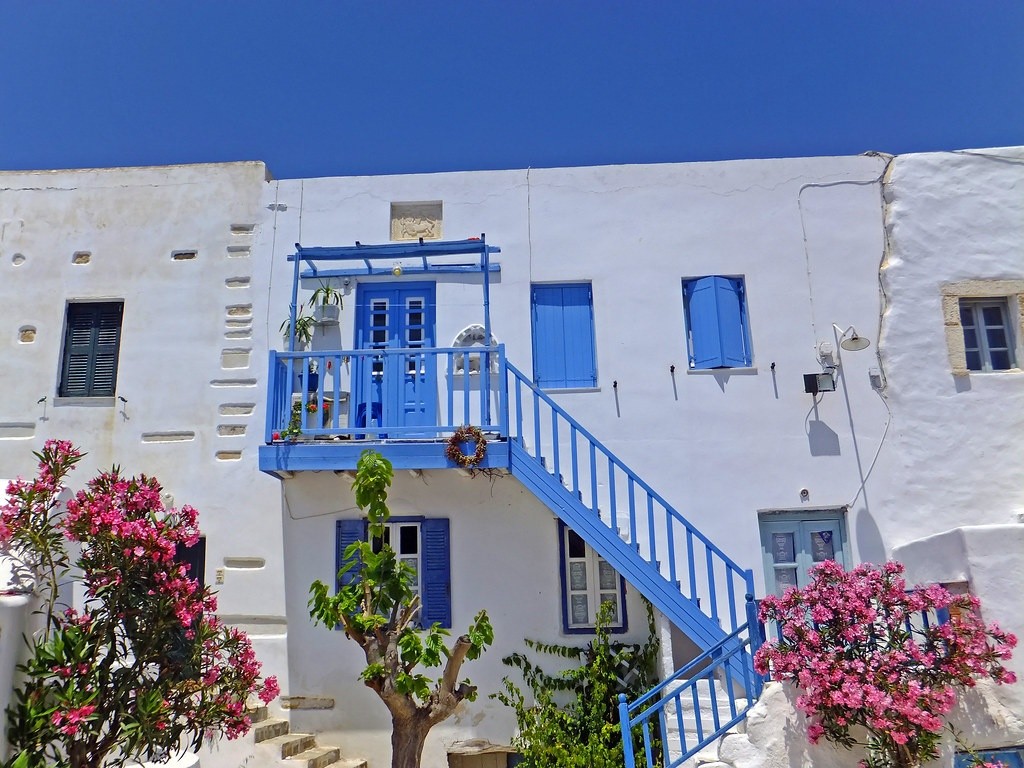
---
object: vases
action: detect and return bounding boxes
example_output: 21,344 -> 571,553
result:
298,373 -> 319,393
299,413 -> 317,440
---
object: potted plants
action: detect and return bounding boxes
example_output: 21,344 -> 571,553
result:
278,303 -> 316,352
307,278 -> 344,322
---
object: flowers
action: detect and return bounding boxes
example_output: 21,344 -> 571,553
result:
301,360 -> 332,373
273,401 -> 329,441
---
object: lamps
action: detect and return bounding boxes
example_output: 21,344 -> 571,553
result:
831,323 -> 870,352
